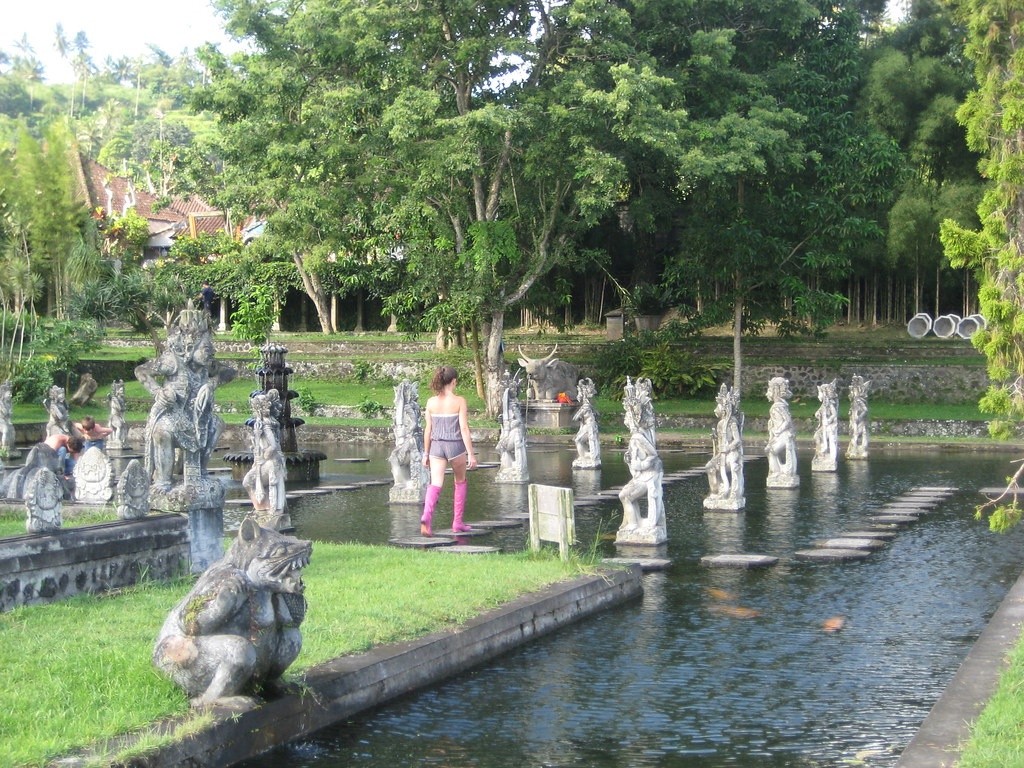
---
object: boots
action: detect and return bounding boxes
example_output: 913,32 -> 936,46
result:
420,484 -> 442,537
453,479 -> 471,533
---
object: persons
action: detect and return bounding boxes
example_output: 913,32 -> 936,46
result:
421,368 -> 478,537
44,435 -> 82,479
75,417 -> 113,452
200,282 -> 215,318
0,311 -> 869,530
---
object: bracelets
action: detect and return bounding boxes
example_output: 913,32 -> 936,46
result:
424,452 -> 430,457
